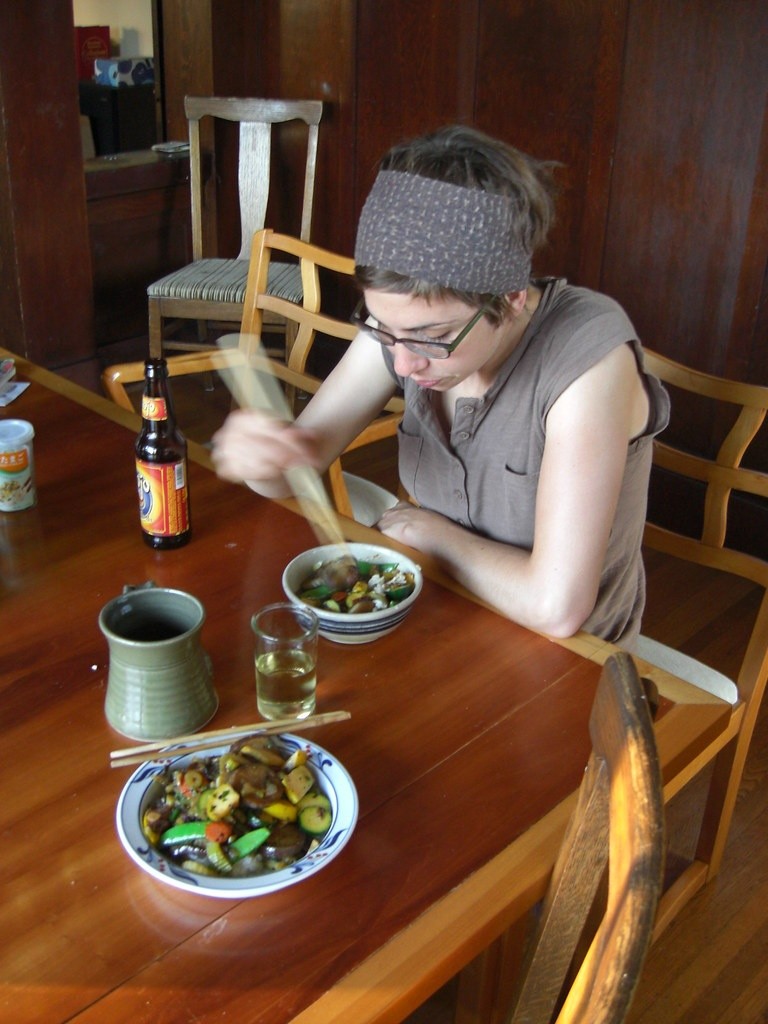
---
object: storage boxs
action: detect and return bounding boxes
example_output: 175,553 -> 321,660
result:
73,25 -> 111,78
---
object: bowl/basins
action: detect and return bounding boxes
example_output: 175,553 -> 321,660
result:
281,540 -> 422,643
115,731 -> 359,898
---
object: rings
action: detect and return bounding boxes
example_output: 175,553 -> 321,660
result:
250,436 -> 282,462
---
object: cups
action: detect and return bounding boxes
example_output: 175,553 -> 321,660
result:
252,602 -> 319,721
99,581 -> 218,744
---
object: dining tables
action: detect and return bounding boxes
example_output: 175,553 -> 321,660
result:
0,348 -> 750,1024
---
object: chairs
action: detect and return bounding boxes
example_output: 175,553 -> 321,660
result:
145,86 -> 325,422
613,349 -> 768,945
494,633 -> 657,1024
104,227 -> 404,530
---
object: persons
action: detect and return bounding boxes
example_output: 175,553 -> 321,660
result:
211,126 -> 670,646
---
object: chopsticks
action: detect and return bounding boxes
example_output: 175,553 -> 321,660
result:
110,710 -> 352,769
209,332 -> 356,574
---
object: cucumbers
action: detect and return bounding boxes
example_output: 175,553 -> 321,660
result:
297,793 -> 332,832
387,585 -> 414,600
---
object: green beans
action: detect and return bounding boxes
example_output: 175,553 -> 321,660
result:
230,827 -> 270,857
359,561 -> 399,575
160,821 -> 209,844
302,586 -> 337,598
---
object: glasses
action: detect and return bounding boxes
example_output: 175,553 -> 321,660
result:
348,296 -> 502,360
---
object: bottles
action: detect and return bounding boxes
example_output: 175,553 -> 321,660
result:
0,418 -> 37,512
135,357 -> 192,549
